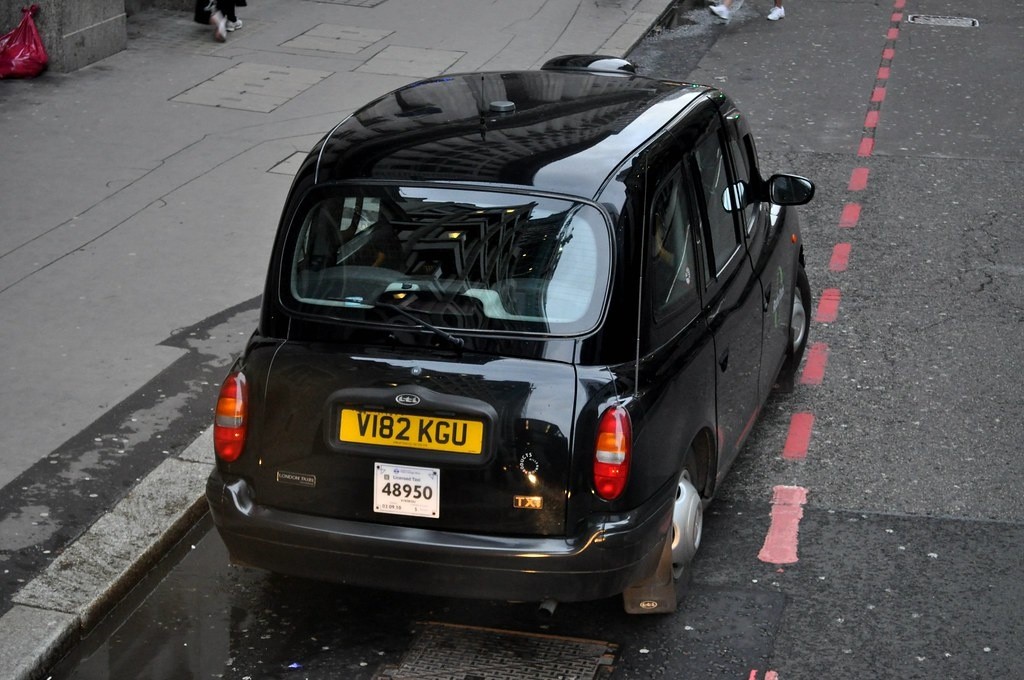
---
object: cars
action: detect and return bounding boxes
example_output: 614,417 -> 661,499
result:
206,54 -> 816,614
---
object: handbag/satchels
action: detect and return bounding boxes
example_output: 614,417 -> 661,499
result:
0,3 -> 48,79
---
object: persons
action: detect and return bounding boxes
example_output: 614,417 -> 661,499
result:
709,0 -> 785,20
194,0 -> 248,41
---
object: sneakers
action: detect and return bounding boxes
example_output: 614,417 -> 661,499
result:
211,11 -> 227,42
767,6 -> 785,20
225,18 -> 244,31
709,5 -> 729,19
